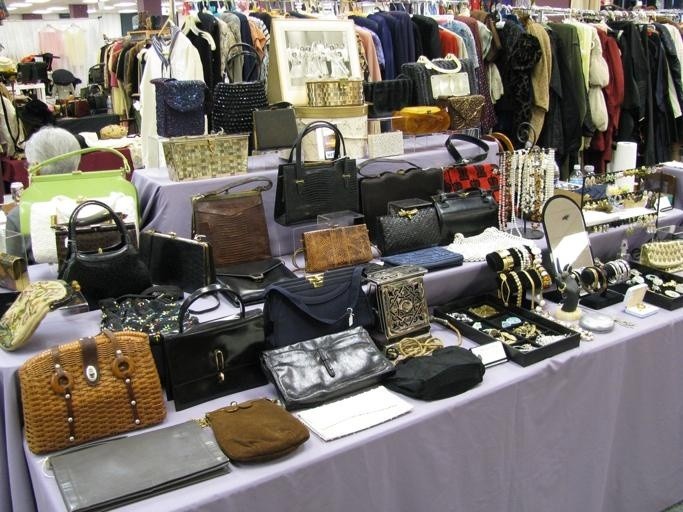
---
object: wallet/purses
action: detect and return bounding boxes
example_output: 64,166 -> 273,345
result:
383,245 -> 463,271
46,417 -> 232,512
0,278 -> 81,352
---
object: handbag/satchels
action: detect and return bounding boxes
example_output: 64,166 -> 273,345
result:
164,285 -> 269,413
211,257 -> 297,307
251,101 -> 300,151
190,177 -> 275,268
68,61 -> 108,119
149,78 -> 206,137
290,223 -> 374,274
258,325 -> 397,412
359,53 -> 511,256
0,145 -> 213,312
264,266 -> 386,349
272,119 -> 359,228
381,344 -> 485,402
17,330 -> 167,455
640,240 -> 683,274
204,397 -> 309,466
368,264 -> 430,343
210,44 -> 271,134
98,292 -> 199,342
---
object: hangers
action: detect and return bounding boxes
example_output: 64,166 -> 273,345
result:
114,1 -> 471,51
495,4 -> 681,34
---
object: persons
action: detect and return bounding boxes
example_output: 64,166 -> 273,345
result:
0,83 -> 82,175
285,37 -> 351,82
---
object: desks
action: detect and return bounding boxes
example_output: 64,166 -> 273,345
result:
3,83 -> 47,103
53,113 -> 121,139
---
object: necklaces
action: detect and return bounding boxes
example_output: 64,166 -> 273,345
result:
498,148 -> 554,238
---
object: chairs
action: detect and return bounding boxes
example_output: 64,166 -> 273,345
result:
86,63 -> 110,86
51,69 -> 80,104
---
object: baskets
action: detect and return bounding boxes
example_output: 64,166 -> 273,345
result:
160,135 -> 250,185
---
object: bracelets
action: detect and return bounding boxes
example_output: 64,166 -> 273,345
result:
496,244 -> 631,312
